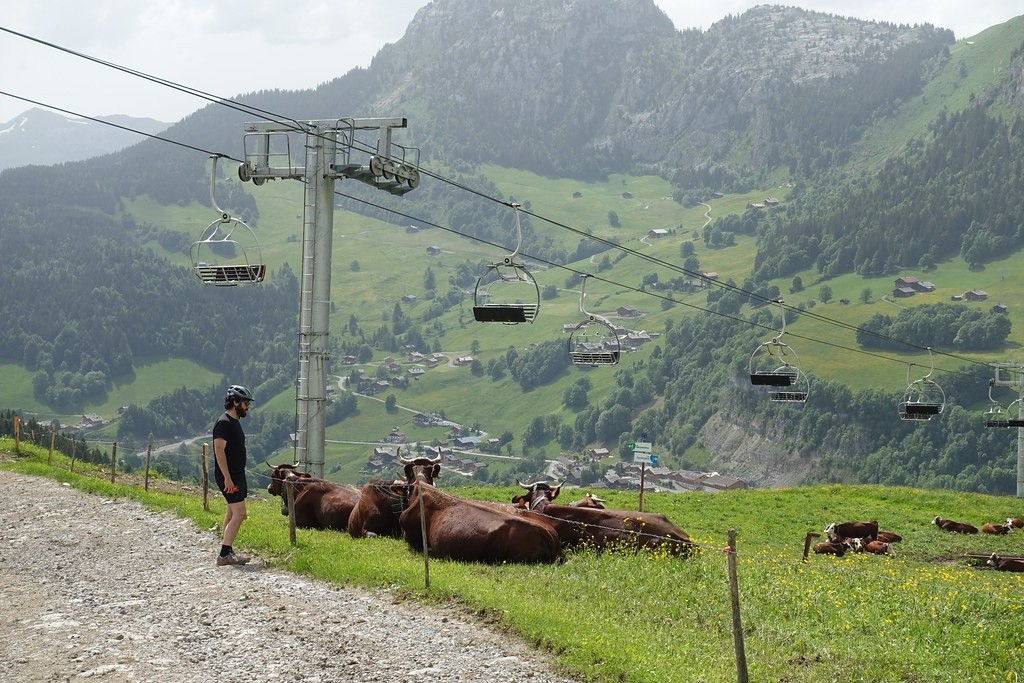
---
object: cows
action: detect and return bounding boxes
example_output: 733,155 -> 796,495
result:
265,446 -> 700,565
932,515 -> 979,535
983,516 -> 1024,536
813,519 -> 901,557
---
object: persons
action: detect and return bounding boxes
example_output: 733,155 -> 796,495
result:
213,384 -> 256,565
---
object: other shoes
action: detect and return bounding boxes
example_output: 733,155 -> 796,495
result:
217,550 -> 251,566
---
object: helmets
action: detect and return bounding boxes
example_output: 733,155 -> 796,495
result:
227,385 -> 255,401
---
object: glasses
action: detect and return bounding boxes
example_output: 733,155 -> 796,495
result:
238,398 -> 250,406
